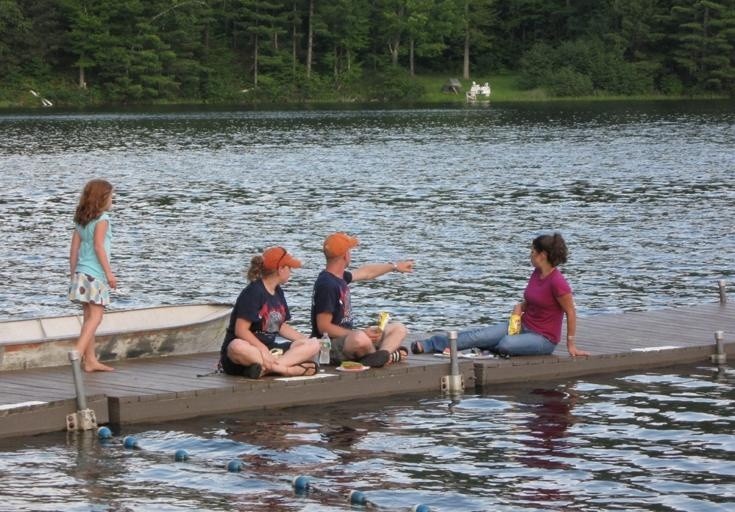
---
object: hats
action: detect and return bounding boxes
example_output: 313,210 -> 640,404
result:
323,233 -> 359,259
260,246 -> 303,274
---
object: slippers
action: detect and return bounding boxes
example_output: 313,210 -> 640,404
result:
292,361 -> 320,377
391,345 -> 408,364
241,362 -> 263,380
410,341 -> 425,354
359,351 -> 390,367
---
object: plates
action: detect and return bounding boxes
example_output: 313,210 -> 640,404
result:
336,365 -> 370,371
434,352 -> 494,360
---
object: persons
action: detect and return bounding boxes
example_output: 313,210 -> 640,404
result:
308,232 -> 416,371
75,432 -> 133,512
235,403 -> 417,512
68,177 -> 118,373
513,381 -> 580,505
408,233 -> 592,357
217,244 -> 322,379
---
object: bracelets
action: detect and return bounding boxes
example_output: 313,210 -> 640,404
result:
386,260 -> 398,272
568,334 -> 577,341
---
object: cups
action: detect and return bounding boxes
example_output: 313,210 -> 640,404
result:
366,325 -> 381,344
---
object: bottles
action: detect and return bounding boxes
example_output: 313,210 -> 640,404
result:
319,333 -> 330,364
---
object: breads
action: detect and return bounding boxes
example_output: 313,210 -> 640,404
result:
379,311 -> 389,331
